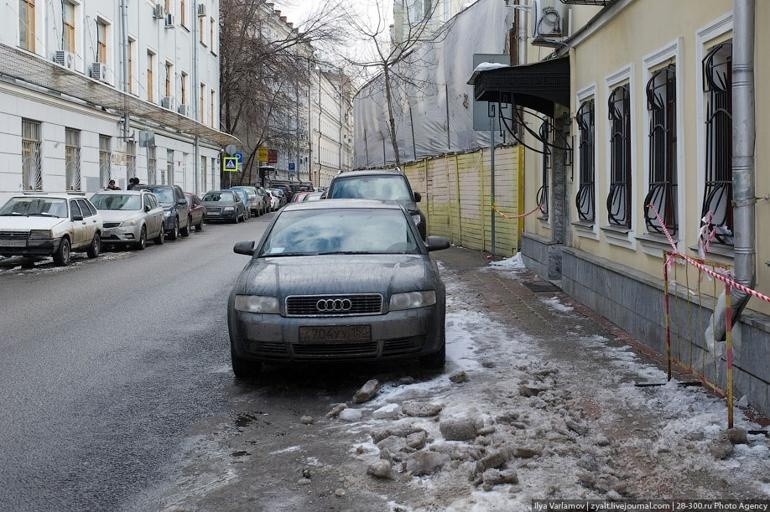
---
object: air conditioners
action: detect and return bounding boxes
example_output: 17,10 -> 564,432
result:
90,62 -> 114,88
55,50 -> 75,75
155,3 -> 176,28
198,3 -> 207,17
531,0 -> 569,37
162,97 -> 192,119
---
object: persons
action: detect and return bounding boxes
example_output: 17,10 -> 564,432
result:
107,180 -> 121,190
127,177 -> 135,190
130,178 -> 140,190
